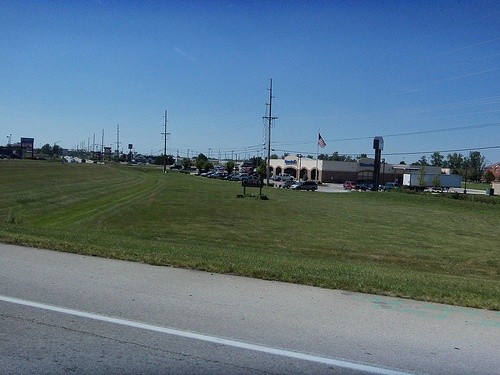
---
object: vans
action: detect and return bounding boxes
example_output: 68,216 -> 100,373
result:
290,180 -> 318,192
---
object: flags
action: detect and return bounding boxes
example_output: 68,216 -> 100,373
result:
318,133 -> 327,149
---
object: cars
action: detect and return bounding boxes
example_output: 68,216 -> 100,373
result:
167,167 -> 304,193
343,178 -> 404,191
